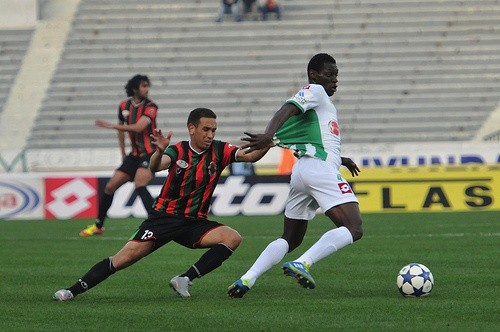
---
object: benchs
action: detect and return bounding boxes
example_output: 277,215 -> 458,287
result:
0,0 -> 500,144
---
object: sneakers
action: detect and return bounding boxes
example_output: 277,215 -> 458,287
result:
168,275 -> 191,298
283,260 -> 315,290
52,288 -> 71,301
226,277 -> 251,298
79,224 -> 105,237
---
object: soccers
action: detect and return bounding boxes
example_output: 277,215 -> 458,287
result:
397,263 -> 434,298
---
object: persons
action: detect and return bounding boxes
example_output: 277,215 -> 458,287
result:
227,53 -> 364,296
80,74 -> 159,237
215,0 -> 281,22
52,108 -> 275,301
